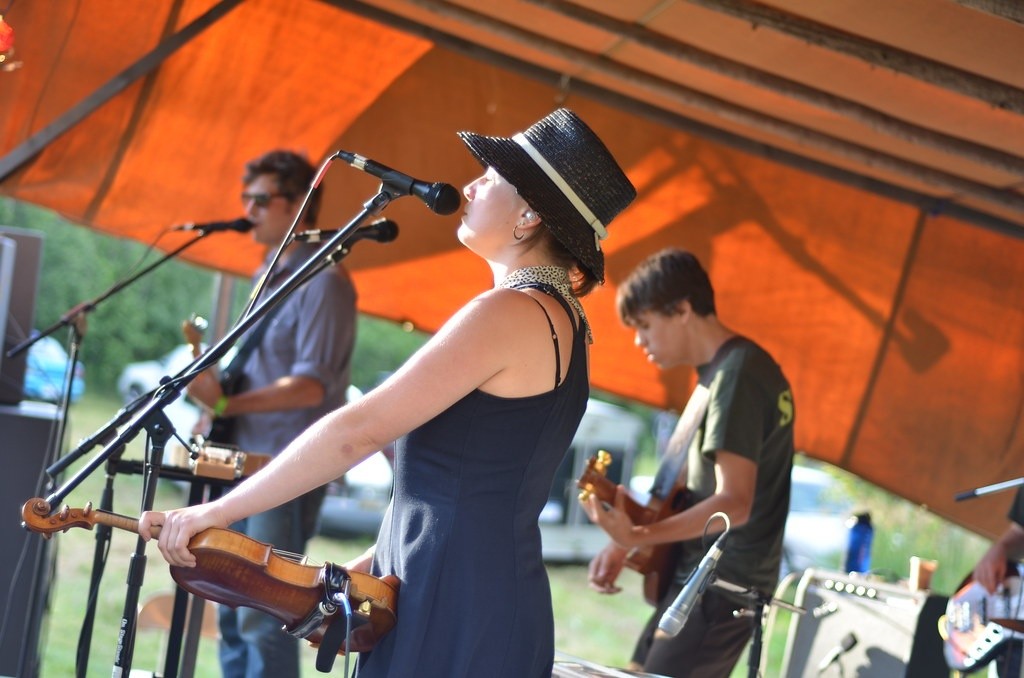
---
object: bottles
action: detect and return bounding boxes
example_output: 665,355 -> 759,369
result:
844,512 -> 874,572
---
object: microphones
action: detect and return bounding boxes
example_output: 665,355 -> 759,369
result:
174,217 -> 254,234
292,217 -> 399,244
819,631 -> 858,671
658,529 -> 731,636
336,150 -> 461,217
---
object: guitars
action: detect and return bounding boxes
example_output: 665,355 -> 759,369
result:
575,447 -> 687,609
940,554 -> 1024,673
177,306 -> 251,473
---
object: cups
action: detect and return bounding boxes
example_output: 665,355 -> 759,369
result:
910,555 -> 939,591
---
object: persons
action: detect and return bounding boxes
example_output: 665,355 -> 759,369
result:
135,106 -> 638,678
963,472 -> 1023,613
576,244 -> 795,678
157,145 -> 358,678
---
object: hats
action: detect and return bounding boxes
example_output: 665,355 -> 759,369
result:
455,108 -> 637,282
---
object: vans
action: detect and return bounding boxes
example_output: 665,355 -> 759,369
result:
540,398 -> 640,565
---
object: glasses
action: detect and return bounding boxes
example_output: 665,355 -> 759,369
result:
241,191 -> 285,206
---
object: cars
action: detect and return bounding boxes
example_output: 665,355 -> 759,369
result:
21,330 -> 83,405
776,466 -> 859,584
120,346 -> 395,536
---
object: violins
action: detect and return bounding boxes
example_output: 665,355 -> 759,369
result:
20,496 -> 402,658
